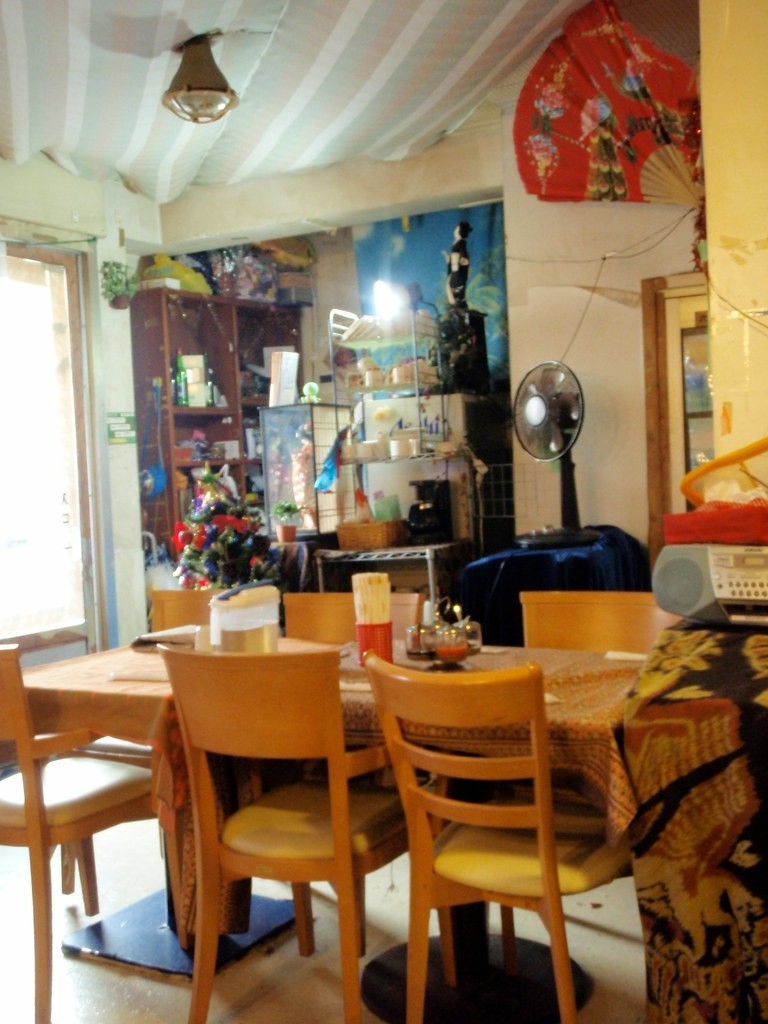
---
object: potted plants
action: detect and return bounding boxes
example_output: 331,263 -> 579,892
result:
101,260 -> 138,311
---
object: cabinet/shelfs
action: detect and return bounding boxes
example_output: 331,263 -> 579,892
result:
131,287 -> 501,596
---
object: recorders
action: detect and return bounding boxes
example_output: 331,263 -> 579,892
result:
652,543 -> 768,629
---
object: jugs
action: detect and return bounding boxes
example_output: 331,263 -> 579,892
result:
209,579 -> 280,652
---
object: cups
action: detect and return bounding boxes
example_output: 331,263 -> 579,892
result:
391,440 -> 405,457
366,371 -> 380,387
340,444 -> 354,460
410,438 -> 421,455
392,367 -> 405,384
355,443 -> 372,459
406,621 -> 481,657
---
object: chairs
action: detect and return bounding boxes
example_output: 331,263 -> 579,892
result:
283,591 -> 424,957
364,650 -> 631,1024
1,646 -> 166,1023
494,588 -> 684,977
3,629 -> 166,895
156,641 -> 458,1024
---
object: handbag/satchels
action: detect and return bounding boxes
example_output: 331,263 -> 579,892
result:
139,465 -> 167,498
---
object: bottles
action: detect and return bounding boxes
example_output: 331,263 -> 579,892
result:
204,350 -> 213,406
175,347 -> 188,406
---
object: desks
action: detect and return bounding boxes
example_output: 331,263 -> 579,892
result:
625,619 -> 768,1024
20,634 -> 642,1024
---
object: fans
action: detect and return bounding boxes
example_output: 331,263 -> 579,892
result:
513,361 -> 601,552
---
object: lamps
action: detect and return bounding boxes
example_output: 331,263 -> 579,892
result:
375,282 -> 440,316
162,36 -> 239,123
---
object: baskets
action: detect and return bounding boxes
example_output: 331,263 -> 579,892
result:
336,518 -> 407,549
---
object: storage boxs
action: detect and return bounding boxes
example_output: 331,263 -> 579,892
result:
277,273 -> 314,305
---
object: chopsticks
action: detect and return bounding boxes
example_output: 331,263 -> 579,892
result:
352,573 -> 389,665
